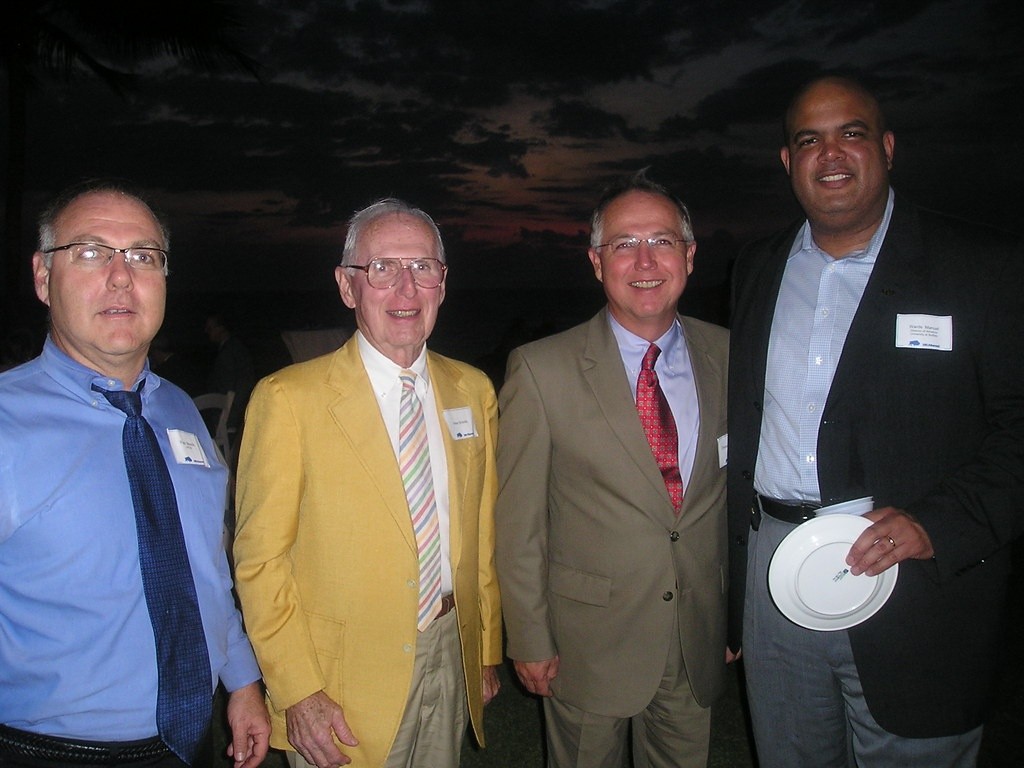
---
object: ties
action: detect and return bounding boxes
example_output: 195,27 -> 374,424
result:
636,343 -> 684,517
91,377 -> 214,764
398,368 -> 442,633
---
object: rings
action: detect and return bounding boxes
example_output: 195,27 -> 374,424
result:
887,535 -> 895,547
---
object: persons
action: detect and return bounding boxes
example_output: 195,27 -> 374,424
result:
726,69 -> 1023,768
0,178 -> 273,768
232,197 -> 503,767
494,179 -> 742,767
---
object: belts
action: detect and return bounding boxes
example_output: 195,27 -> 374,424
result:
435,593 -> 455,620
753,489 -> 823,525
1,689 -> 220,760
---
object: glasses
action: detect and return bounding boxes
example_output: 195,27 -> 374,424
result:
45,242 -> 168,272
598,236 -> 688,255
347,257 -> 449,290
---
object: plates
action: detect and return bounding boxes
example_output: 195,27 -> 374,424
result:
768,514 -> 898,632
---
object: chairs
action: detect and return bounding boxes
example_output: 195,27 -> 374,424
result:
279,327 -> 348,367
192,390 -> 237,463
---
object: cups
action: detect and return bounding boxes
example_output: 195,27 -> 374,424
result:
813,496 -> 874,516
